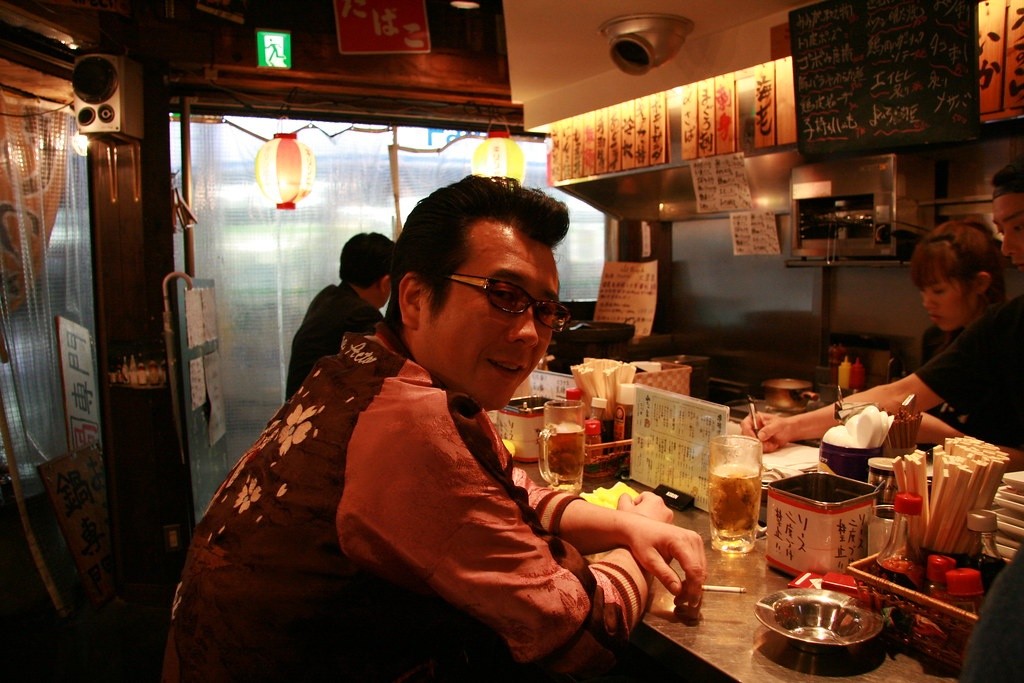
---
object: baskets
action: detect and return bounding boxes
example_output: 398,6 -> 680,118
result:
630,361 -> 693,398
583,439 -> 634,477
849,544 -> 1009,672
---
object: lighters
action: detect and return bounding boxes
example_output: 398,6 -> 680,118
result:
821,572 -> 858,598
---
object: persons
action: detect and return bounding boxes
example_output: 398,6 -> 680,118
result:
957,541 -> 1024,683
160,174 -> 707,683
739,157 -> 1023,472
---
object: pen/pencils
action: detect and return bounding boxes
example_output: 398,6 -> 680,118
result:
746,394 -> 758,437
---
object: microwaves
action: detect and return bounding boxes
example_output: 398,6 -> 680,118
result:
789,154 -> 936,257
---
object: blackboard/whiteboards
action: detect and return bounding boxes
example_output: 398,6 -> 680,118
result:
788,1 -> 981,157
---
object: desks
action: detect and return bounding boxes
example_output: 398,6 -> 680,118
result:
516,461 -> 958,683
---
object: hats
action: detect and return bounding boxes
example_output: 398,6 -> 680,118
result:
992,155 -> 1024,198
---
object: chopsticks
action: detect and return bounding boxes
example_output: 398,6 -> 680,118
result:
882,407 -> 1011,555
570,357 -> 636,419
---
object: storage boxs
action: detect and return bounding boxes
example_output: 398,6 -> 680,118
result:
766,470 -> 882,580
497,396 -> 558,462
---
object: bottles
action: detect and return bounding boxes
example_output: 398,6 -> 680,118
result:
838,356 -> 865,391
590,397 -> 609,444
944,567 -> 983,618
924,554 -> 956,603
867,458 -> 906,505
870,492 -> 924,593
108,352 -> 169,385
614,383 -> 635,441
566,389 -> 582,400
962,510 -> 1007,582
584,420 -> 602,472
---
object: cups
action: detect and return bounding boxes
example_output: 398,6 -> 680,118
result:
538,399 -> 585,492
868,505 -> 896,556
708,435 -> 763,553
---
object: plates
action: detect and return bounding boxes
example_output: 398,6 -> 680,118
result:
984,470 -> 1024,560
753,589 -> 885,654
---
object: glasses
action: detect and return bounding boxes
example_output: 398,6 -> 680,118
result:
444,273 -> 572,336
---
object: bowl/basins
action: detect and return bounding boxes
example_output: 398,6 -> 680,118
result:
818,442 -> 883,483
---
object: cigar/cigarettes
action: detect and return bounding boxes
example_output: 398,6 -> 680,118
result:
700,585 -> 747,593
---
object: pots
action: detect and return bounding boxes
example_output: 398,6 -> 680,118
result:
762,378 -> 818,407
551,317 -> 636,340
